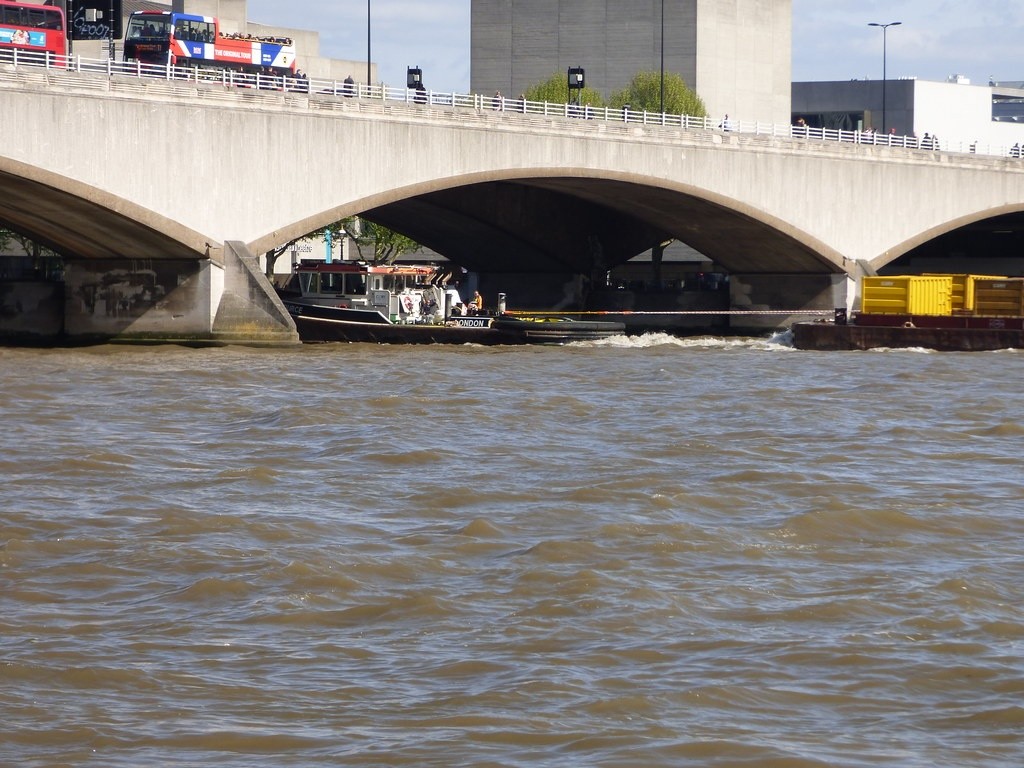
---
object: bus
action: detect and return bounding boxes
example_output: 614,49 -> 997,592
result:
122,10 -> 296,91
0,0 -> 68,72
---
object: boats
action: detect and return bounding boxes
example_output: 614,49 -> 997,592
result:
273,259 -> 627,345
792,273 -> 1023,351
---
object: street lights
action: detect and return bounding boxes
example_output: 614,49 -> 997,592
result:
338,222 -> 345,259
867,20 -> 902,133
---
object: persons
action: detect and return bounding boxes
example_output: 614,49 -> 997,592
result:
796,118 -> 805,138
517,94 -> 526,113
1010,143 -> 1024,158
569,97 -> 581,118
470,291 -> 483,316
970,140 -> 977,154
719,113 -> 731,132
133,24 -> 309,93
583,103 -> 594,119
413,84 -> 427,104
492,91 -> 501,111
622,102 -> 632,123
344,76 -> 354,98
863,127 -> 939,152
